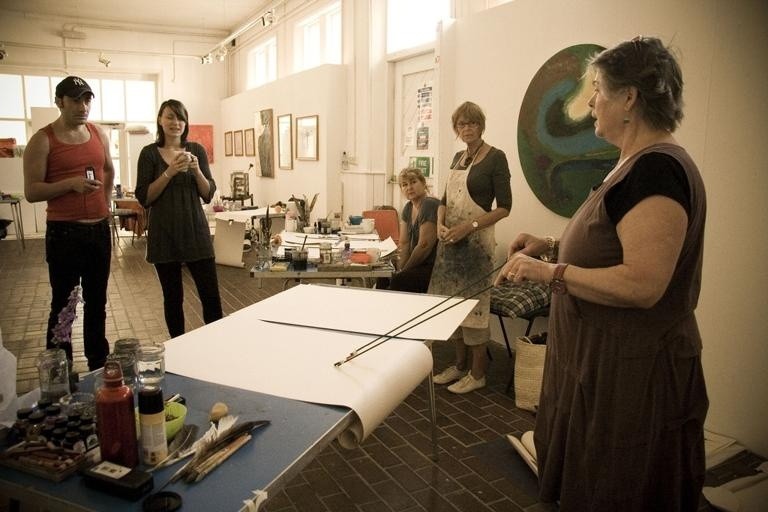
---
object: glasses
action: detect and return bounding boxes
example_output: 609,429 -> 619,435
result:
456,122 -> 478,129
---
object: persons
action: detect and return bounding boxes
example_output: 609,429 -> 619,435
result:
375,167 -> 441,292
23,75 -> 115,375
493,36 -> 708,512
134,98 -> 223,339
426,100 -> 512,395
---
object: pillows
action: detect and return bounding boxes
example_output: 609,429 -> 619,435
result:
489,279 -> 552,319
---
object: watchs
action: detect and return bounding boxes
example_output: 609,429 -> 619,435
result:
550,261 -> 568,297
472,218 -> 480,230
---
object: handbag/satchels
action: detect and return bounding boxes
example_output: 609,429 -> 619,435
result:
514,331 -> 546,412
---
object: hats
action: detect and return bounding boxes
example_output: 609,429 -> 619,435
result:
56,76 -> 95,98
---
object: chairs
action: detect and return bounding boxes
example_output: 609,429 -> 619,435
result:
485,310 -> 550,394
109,209 -> 147,246
361,210 -> 400,289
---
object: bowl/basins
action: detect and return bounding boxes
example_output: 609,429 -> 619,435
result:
350,216 -> 363,225
134,401 -> 188,443
303,227 -> 316,234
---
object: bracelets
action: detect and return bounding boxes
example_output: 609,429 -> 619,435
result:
162,169 -> 173,180
543,235 -> 556,258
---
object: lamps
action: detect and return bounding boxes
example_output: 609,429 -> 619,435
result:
0,41 -> 113,67
201,0 -> 283,65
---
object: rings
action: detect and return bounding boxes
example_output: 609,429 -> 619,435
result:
507,271 -> 516,276
450,238 -> 453,242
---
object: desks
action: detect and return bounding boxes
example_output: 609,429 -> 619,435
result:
0,193 -> 26,252
110,198 -> 149,247
217,212 -> 285,252
252,264 -> 396,291
0,281 -> 467,512
221,194 -> 254,207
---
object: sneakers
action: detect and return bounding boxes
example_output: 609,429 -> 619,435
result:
433,365 -> 470,385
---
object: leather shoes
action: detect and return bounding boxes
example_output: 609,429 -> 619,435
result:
447,370 -> 487,395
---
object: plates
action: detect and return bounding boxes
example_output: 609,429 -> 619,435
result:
318,215 -> 340,234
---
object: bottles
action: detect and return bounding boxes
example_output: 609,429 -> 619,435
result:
37,348 -> 70,400
320,246 -> 332,264
95,363 -> 167,469
341,242 -> 353,268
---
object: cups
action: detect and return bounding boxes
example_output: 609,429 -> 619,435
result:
105,338 -> 165,386
291,248 -> 309,270
362,219 -> 375,234
174,151 -> 191,173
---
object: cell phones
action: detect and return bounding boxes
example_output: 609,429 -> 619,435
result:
85,167 -> 95,181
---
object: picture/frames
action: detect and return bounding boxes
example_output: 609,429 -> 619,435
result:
277,113 -> 293,169
234,130 -> 244,156
244,128 -> 256,156
295,114 -> 319,162
224,131 -> 233,156
